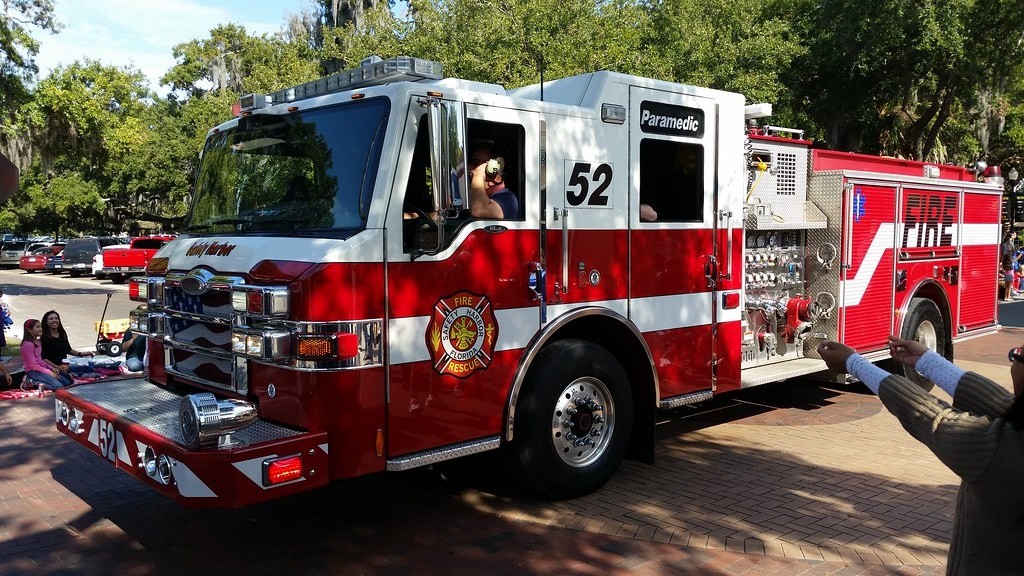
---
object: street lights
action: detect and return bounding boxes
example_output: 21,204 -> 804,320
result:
1008,168 -> 1019,232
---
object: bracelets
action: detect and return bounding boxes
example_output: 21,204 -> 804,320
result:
78,352 -> 82,356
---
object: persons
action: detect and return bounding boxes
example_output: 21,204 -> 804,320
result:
402,140 -> 519,220
0,347 -> 12,385
817,334 -> 1024,576
122,304 -> 150,371
0,290 -> 13,347
39,310 -> 94,385
998,230 -> 1024,301
20,319 -> 74,392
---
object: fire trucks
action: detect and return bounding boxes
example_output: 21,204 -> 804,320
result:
52,49 -> 1006,512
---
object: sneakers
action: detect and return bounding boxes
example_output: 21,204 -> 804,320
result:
20,374 -> 34,392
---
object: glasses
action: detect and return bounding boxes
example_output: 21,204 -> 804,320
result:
468,159 -> 489,166
1008,347 -> 1024,363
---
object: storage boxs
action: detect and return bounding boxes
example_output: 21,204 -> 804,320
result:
95,318 -> 130,333
99,332 -> 127,341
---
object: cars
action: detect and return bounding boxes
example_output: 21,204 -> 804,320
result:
0,231 -> 179,285
